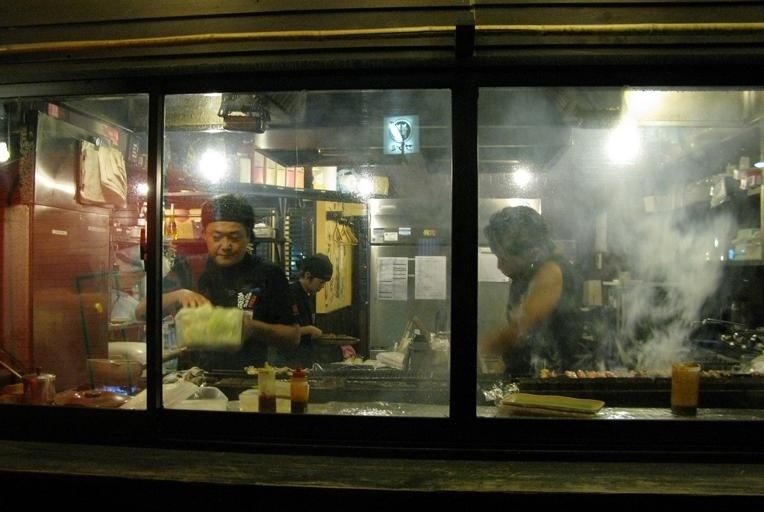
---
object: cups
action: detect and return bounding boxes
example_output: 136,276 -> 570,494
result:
670,364 -> 701,417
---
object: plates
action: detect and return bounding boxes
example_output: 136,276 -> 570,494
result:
500,392 -> 606,416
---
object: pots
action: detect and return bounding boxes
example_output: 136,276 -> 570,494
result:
85,346 -> 190,385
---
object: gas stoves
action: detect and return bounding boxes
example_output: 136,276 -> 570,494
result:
59,379 -> 148,409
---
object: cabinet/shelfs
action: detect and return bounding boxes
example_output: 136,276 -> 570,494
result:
622,108 -> 764,364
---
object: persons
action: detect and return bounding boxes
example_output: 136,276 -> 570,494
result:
261,253 -> 334,371
477,204 -> 590,379
132,192 -> 302,400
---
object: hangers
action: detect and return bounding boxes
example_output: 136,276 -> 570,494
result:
332,216 -> 358,245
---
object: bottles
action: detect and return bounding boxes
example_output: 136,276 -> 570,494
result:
289,365 -> 310,414
23,366 -> 57,404
164,203 -> 206,240
256,361 -> 277,412
